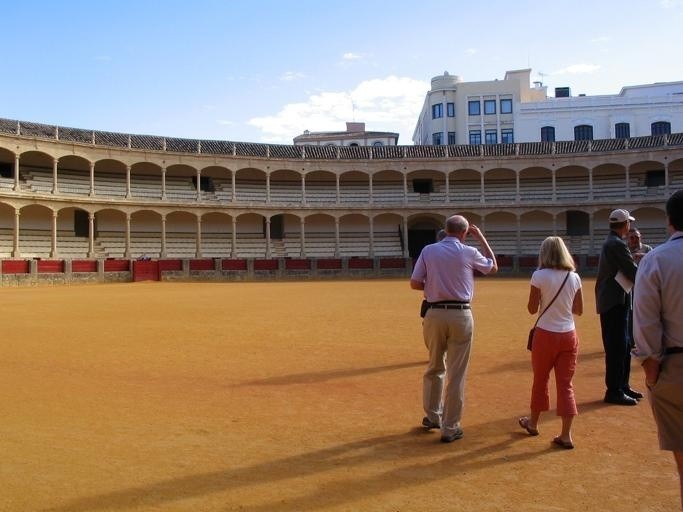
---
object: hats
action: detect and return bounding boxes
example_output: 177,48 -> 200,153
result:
608,209 -> 635,222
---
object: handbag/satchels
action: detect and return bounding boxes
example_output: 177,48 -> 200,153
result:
527,328 -> 534,351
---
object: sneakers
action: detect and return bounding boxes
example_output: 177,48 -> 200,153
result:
422,417 -> 441,428
440,430 -> 463,442
519,417 -> 539,435
554,435 -> 574,449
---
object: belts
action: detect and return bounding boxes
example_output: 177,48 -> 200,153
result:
662,347 -> 683,355
428,304 -> 471,309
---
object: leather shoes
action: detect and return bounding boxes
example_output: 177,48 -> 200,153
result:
622,387 -> 643,398
604,389 -> 637,404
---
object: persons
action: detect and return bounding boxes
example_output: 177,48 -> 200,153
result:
518,236 -> 583,450
433,229 -> 446,243
631,186 -> 682,490
624,227 -> 653,355
591,207 -> 644,406
407,215 -> 499,444
